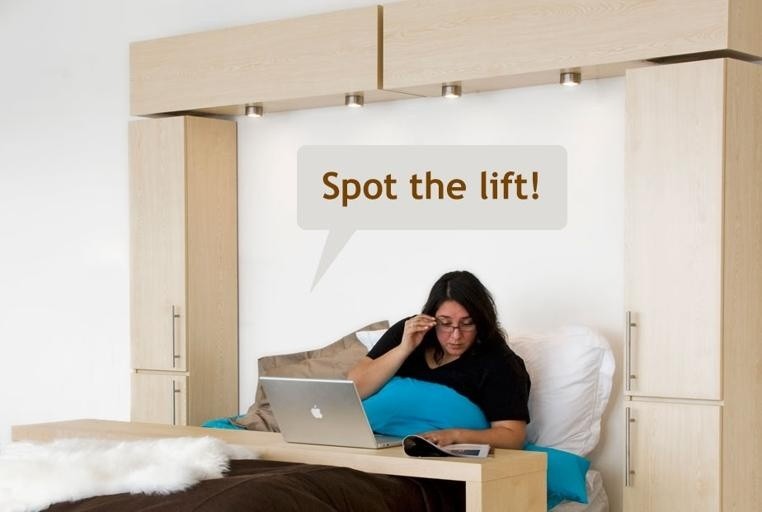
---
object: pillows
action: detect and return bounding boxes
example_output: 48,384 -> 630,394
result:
227,318 -> 388,432
354,326 -> 616,456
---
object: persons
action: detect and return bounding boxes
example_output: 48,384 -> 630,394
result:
348,271 -> 531,448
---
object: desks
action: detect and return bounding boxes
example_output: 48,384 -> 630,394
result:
9,418 -> 547,512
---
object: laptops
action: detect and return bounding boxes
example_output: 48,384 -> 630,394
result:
258,376 -> 402,449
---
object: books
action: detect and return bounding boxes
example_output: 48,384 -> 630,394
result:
401,435 -> 492,459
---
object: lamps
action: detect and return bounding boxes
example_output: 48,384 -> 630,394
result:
560,73 -> 581,85
245,106 -> 262,118
345,96 -> 363,108
443,86 -> 461,97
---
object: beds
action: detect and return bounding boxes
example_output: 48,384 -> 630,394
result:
1,414 -> 613,512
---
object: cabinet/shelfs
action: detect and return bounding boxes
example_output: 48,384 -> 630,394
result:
127,114 -> 238,427
621,57 -> 761,511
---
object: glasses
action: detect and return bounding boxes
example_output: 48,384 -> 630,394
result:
433,324 -> 475,333
